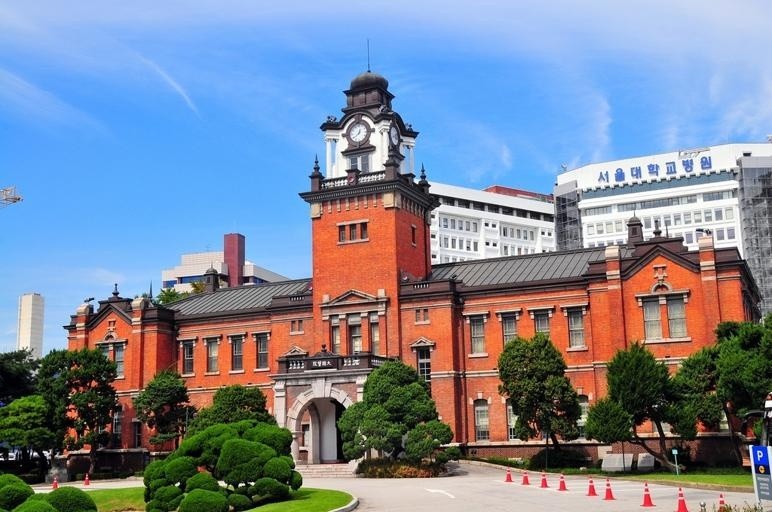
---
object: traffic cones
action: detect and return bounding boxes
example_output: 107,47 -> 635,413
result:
539,471 -> 548,487
84,473 -> 90,484
504,467 -> 513,481
717,494 -> 726,511
675,488 -> 687,512
557,474 -> 567,490
522,470 -> 529,483
52,477 -> 58,489
641,483 -> 655,506
587,477 -> 615,500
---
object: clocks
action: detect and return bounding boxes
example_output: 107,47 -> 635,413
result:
342,115 -> 374,147
